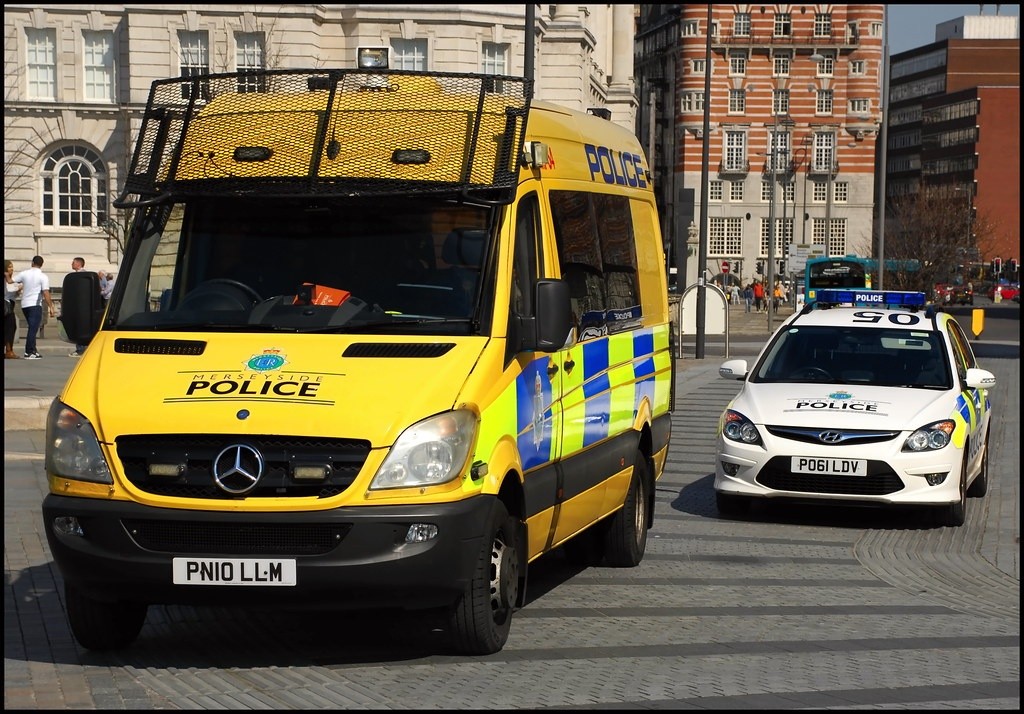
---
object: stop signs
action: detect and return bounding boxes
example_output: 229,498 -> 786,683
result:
722,261 -> 729,273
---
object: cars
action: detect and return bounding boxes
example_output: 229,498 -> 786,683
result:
713,289 -> 997,527
936,283 -> 973,305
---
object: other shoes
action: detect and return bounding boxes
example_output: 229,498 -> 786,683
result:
5,353 -> 20,359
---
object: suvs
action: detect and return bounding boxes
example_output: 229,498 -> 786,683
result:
988,285 -> 1021,303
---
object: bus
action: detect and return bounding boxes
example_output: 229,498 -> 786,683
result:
804,254 -> 871,307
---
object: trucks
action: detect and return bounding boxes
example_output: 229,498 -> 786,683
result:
42,71 -> 679,656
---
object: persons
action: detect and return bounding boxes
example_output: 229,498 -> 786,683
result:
716,280 -> 787,315
2,256 -> 121,359
148,283 -> 152,311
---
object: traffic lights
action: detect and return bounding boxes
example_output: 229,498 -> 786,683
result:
756,262 -> 761,274
780,261 -> 785,274
1012,260 -> 1016,271
996,259 -> 1001,272
733,261 -> 738,273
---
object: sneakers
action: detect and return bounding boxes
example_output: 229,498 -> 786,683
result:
24,352 -> 43,360
69,351 -> 80,357
